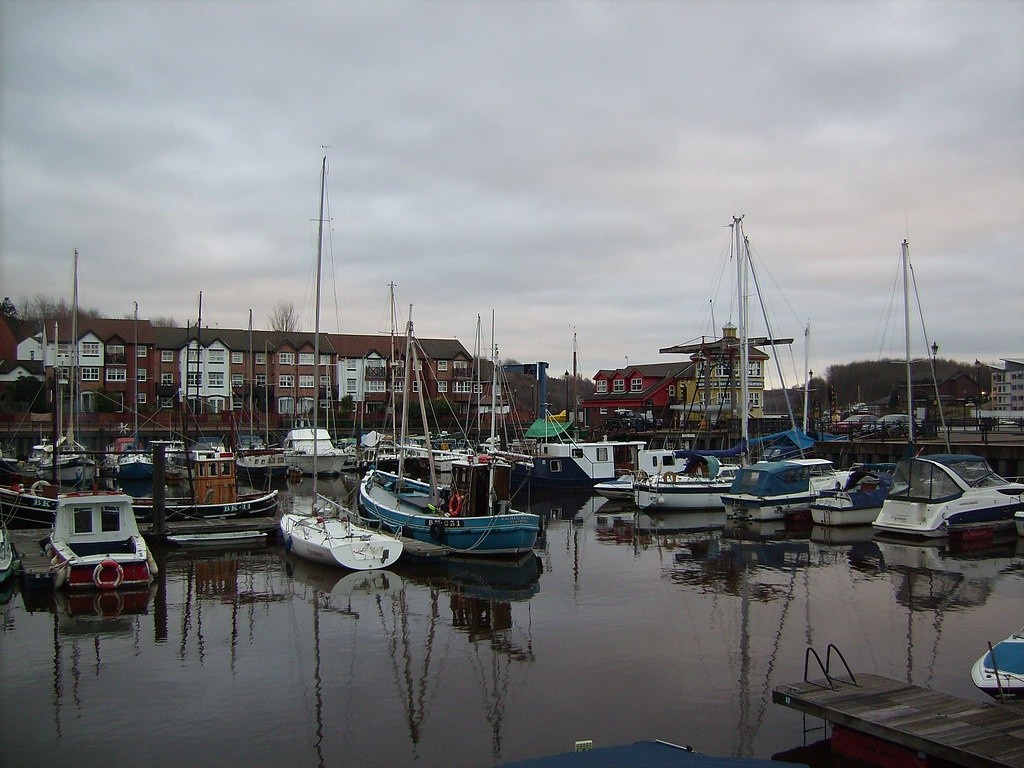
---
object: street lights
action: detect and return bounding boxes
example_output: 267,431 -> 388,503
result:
974,358 -> 981,431
564,369 -> 570,422
809,369 -> 814,418
931,341 -> 939,438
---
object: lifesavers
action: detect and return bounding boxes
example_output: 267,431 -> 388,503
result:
93,590 -> 124,619
30,480 -> 51,497
93,561 -> 125,590
448,493 -> 462,516
664,471 -> 676,483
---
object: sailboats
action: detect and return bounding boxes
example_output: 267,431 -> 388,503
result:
277,144 -> 405,573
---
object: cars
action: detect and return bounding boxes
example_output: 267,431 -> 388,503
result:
828,414 -> 880,435
861,413 -> 920,439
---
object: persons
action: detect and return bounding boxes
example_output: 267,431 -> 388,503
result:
441,482 -> 466,518
403,451 -> 429,484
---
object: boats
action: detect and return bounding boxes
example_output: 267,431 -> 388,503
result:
46,480 -> 158,591
970,626 -> 1024,702
493,737 -> 813,768
0,211 -> 1024,587
358,301 -> 541,556
